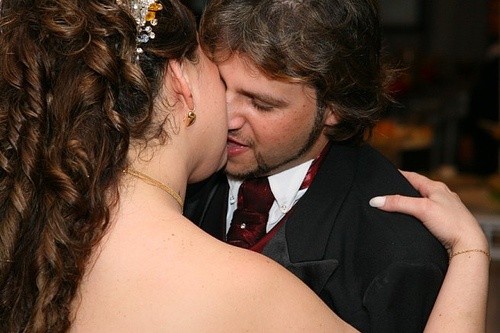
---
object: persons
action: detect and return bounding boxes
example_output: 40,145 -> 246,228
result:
1,0 -> 490,333
182,0 -> 453,333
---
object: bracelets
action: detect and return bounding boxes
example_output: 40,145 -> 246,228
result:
445,248 -> 491,259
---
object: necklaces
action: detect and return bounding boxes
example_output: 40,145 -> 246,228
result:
123,167 -> 184,214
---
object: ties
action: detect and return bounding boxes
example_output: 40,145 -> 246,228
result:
225,173 -> 274,249
